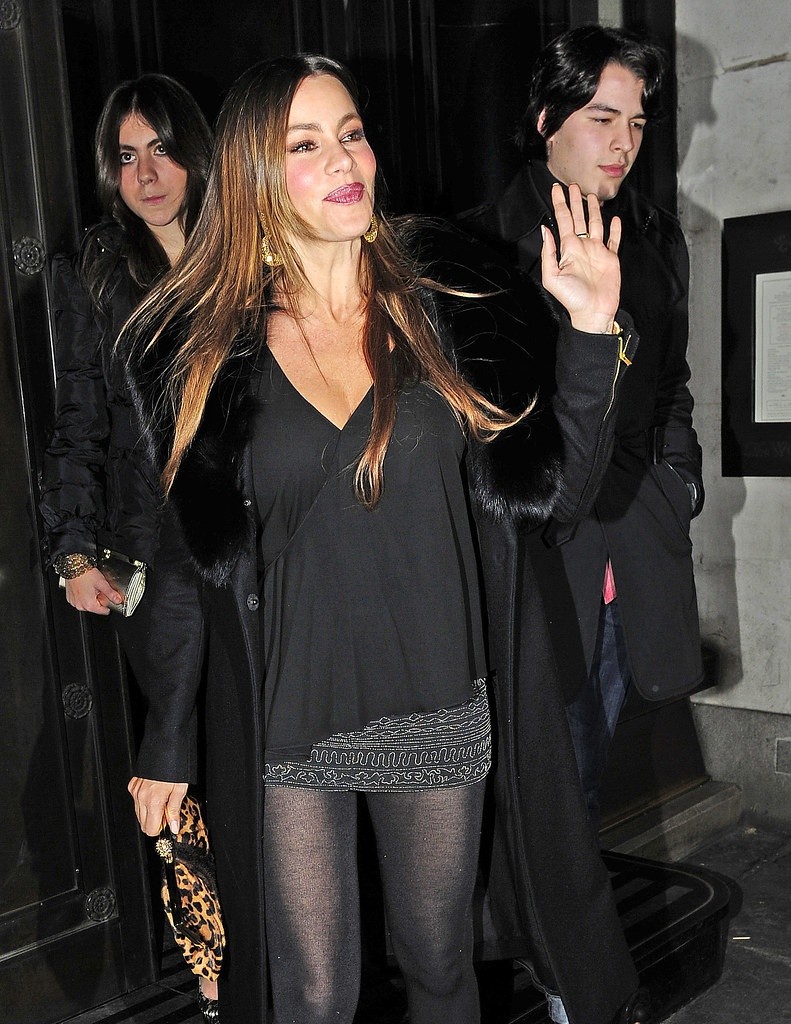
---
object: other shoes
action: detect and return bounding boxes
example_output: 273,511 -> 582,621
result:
197,975 -> 218,1024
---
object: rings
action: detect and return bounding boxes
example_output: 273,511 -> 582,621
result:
577,233 -> 587,236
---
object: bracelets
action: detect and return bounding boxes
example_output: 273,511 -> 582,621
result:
54,554 -> 97,578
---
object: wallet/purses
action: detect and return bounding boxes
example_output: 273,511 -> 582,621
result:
154,794 -> 227,980
58,545 -> 147,617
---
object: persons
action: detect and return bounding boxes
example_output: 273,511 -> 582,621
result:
108,51 -> 640,1024
37,75 -> 213,692
416,25 -> 705,1024
